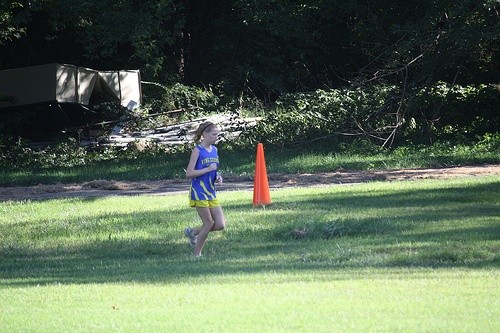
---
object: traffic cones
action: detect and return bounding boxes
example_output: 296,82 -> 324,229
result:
252,142 -> 272,208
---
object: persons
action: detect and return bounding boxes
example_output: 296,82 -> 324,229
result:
184,121 -> 226,258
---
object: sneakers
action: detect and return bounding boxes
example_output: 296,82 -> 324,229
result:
184,228 -> 197,249
